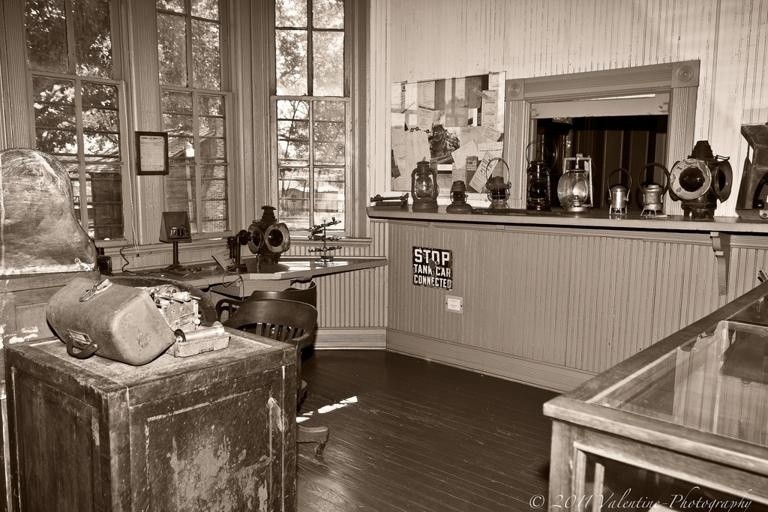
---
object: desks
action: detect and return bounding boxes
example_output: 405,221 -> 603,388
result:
126,253 -> 391,289
541,270 -> 768,511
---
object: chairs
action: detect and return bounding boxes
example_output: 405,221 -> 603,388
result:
210,280 -> 333,459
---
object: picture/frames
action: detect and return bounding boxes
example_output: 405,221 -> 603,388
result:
133,130 -> 170,177
